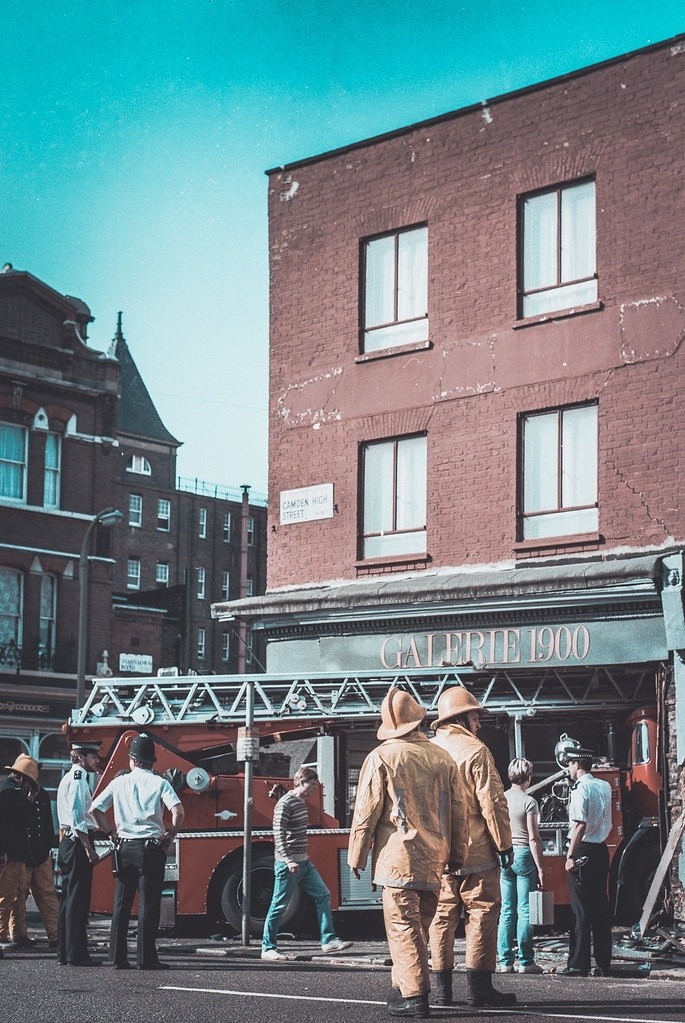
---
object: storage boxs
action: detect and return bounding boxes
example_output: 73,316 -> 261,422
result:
528,884 -> 555,926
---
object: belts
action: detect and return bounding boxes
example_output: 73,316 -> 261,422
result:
122,839 -> 145,842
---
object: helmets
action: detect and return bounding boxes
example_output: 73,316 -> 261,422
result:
377,688 -> 427,741
430,686 -> 485,730
4,756 -> 39,787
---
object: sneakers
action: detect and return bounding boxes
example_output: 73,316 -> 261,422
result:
321,939 -> 353,952
261,949 -> 287,960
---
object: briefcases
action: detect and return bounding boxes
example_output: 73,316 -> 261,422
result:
529,884 -> 555,926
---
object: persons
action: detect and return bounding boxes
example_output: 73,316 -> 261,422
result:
497,757 -> 546,974
347,686 -> 468,1017
0,754 -> 58,947
261,768 -> 353,961
57,740 -> 102,965
430,686 -> 518,1007
558,748 -> 612,974
90,735 -> 185,969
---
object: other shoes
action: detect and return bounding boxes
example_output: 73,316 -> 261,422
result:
501,965 -> 514,972
67,959 -> 102,967
137,962 -> 170,970
556,966 -> 589,976
600,969 -> 611,976
518,963 -> 544,974
11,937 -> 36,946
114,960 -> 130,969
0,936 -> 11,943
57,960 -> 67,965
49,941 -> 57,948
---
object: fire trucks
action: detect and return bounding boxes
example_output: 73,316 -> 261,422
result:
51,672 -> 669,942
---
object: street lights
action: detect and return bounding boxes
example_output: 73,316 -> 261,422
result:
75,505 -> 127,710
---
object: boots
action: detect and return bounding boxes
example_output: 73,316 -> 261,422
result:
388,994 -> 429,1017
467,971 -> 517,1006
435,971 -> 453,1005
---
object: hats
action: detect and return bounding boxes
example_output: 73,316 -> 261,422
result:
128,733 -> 157,763
70,739 -> 103,751
565,747 -> 594,758
